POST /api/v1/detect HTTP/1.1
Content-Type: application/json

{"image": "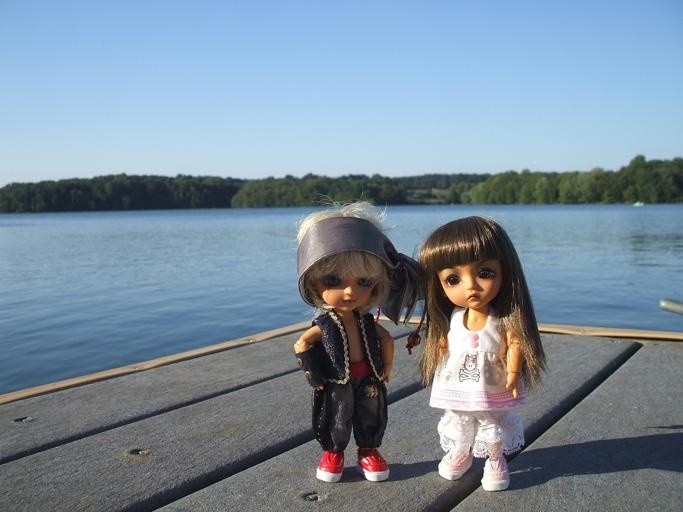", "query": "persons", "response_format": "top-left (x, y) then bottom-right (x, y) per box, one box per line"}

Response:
top-left (412, 215), bottom-right (549, 492)
top-left (293, 201), bottom-right (430, 482)
